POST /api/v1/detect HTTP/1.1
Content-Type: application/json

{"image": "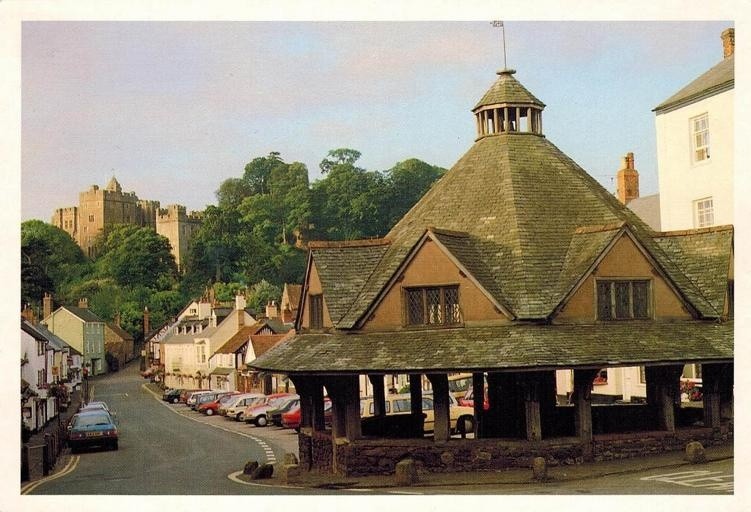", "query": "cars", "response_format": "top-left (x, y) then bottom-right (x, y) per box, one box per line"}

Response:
top-left (68, 402), bottom-right (119, 452)
top-left (422, 373), bottom-right (490, 410)
top-left (281, 397), bottom-right (334, 430)
top-left (359, 396), bottom-right (476, 434)
top-left (161, 390), bottom-right (300, 430)
